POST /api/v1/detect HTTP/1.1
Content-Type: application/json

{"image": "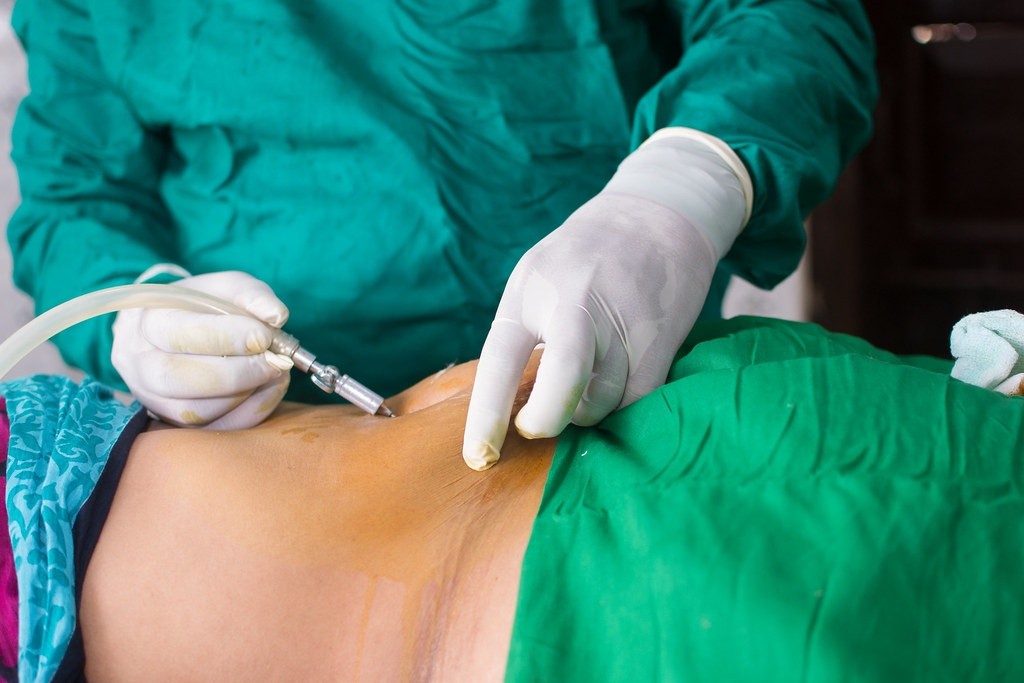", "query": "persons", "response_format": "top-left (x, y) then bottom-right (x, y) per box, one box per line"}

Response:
top-left (6, 0), bottom-right (877, 470)
top-left (0, 316), bottom-right (1024, 683)
